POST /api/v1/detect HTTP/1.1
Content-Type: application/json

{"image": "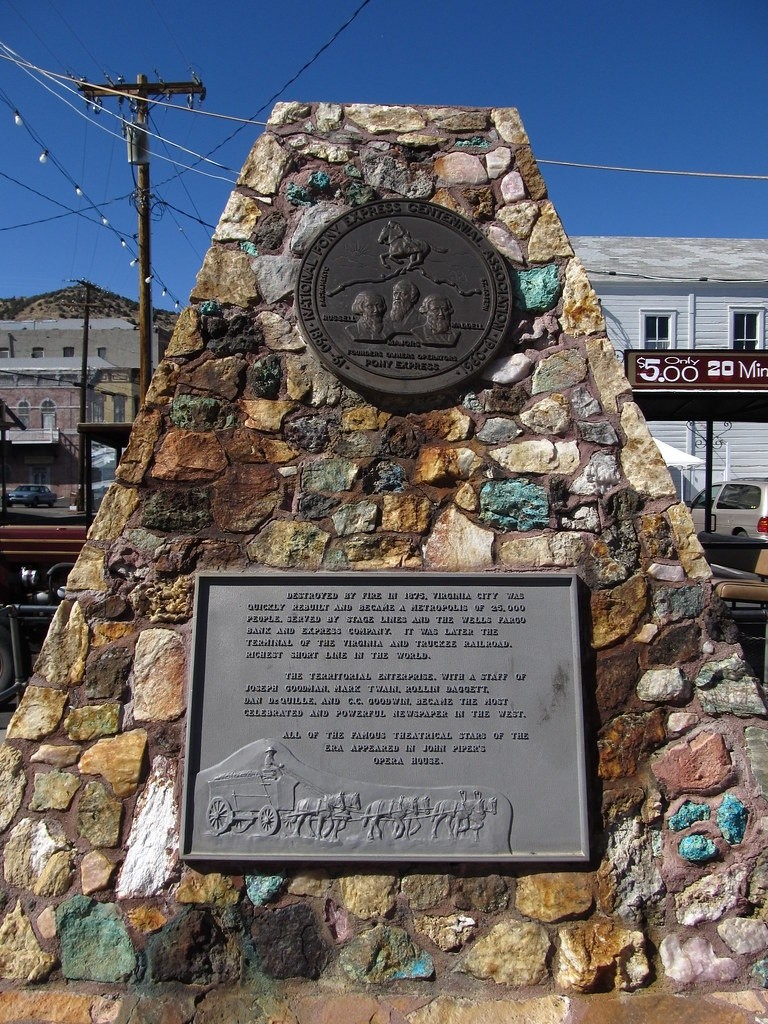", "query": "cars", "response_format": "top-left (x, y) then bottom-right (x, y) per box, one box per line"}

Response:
top-left (6, 484), bottom-right (57, 508)
top-left (684, 481), bottom-right (768, 541)
